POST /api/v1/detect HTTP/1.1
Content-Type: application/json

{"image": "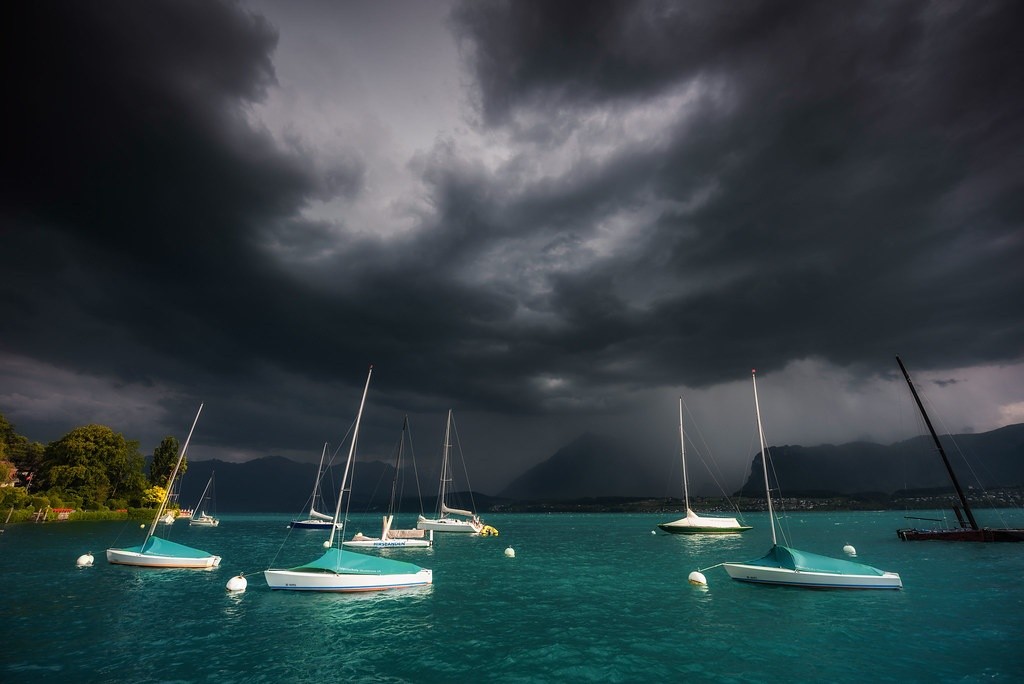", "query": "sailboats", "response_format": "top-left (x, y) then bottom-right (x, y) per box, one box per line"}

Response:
top-left (105, 402), bottom-right (223, 571)
top-left (342, 414), bottom-right (434, 550)
top-left (895, 352), bottom-right (1024, 544)
top-left (290, 442), bottom-right (343, 530)
top-left (190, 470), bottom-right (220, 528)
top-left (657, 396), bottom-right (755, 536)
top-left (263, 364), bottom-right (433, 592)
top-left (417, 408), bottom-right (486, 534)
top-left (722, 368), bottom-right (904, 593)
